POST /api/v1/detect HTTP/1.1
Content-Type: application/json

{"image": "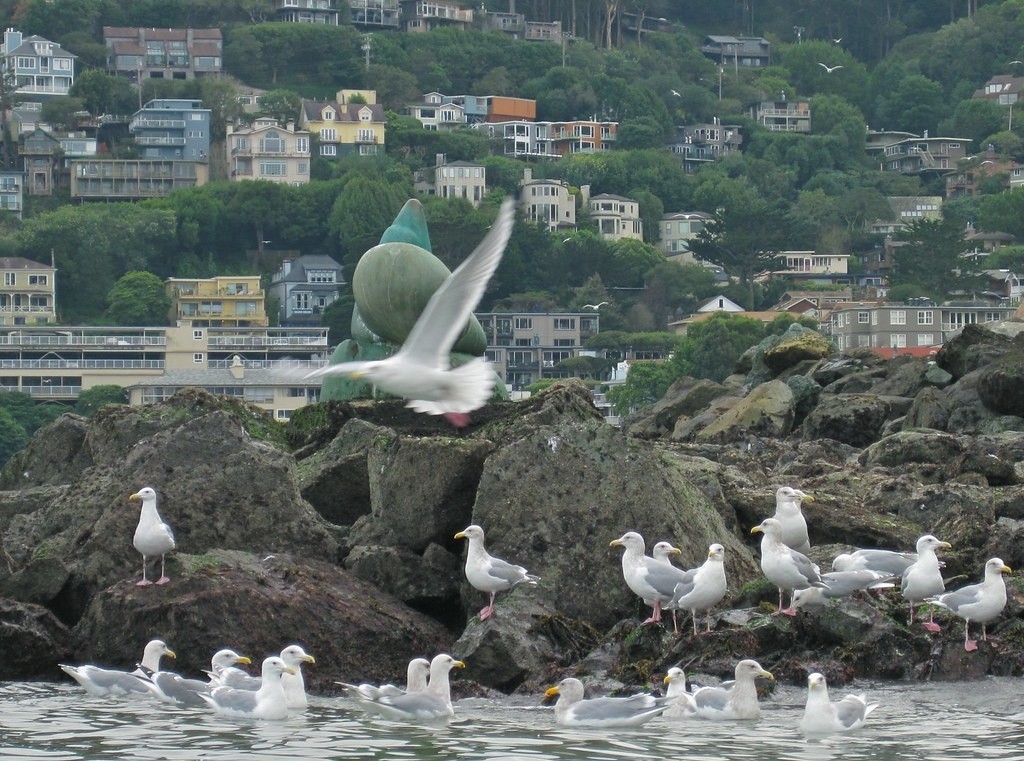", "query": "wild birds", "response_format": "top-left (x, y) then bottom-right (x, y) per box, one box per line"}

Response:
top-left (652, 541), bottom-right (727, 636)
top-left (298, 194), bottom-right (517, 429)
top-left (671, 88), bottom-right (681, 97)
top-left (819, 62), bottom-right (844, 74)
top-left (771, 486), bottom-right (953, 631)
top-left (801, 672), bottom-right (880, 734)
top-left (453, 524), bottom-right (543, 622)
top-left (750, 517), bottom-right (832, 617)
top-left (661, 659), bottom-right (774, 721)
top-left (922, 558), bottom-right (1013, 652)
top-left (544, 677), bottom-right (679, 729)
top-left (832, 37), bottom-right (843, 43)
top-left (608, 531), bottom-right (699, 625)
top-left (129, 486), bottom-right (177, 587)
top-left (58, 639), bottom-right (466, 725)
